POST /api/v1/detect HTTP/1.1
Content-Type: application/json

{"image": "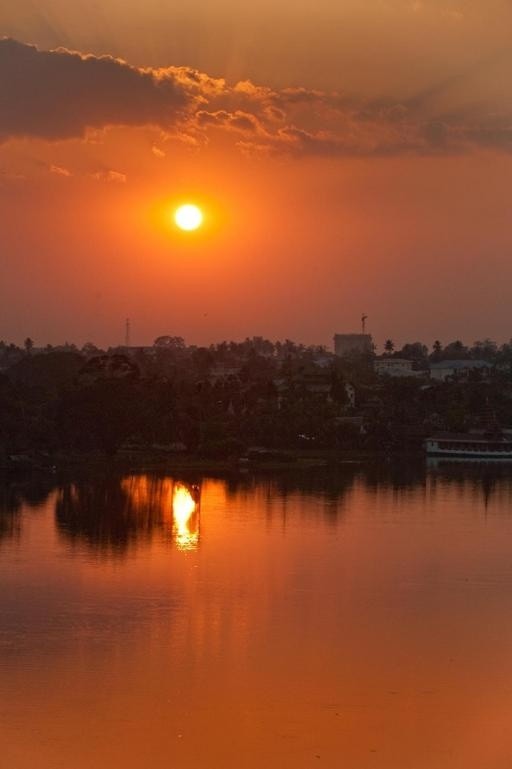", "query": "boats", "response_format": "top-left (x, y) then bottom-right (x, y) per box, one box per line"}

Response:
top-left (422, 432), bottom-right (512, 459)
